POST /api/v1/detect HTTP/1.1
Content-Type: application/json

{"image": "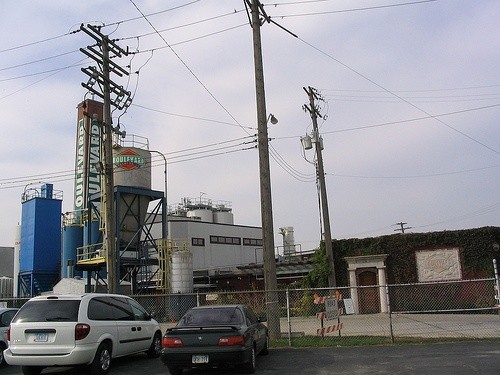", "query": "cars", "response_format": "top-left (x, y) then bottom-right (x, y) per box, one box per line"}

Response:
top-left (0, 308), bottom-right (19, 367)
top-left (159, 303), bottom-right (269, 375)
top-left (3, 294), bottom-right (163, 375)
